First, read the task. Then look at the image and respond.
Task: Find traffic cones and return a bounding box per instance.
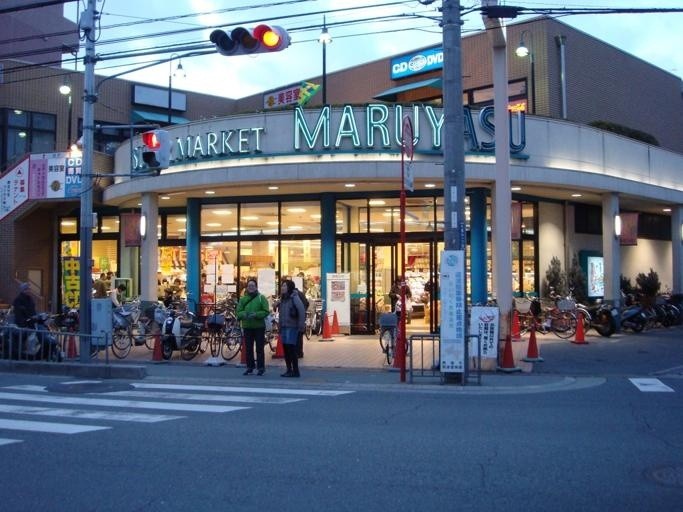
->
[495,334,521,373]
[318,311,336,343]
[569,311,590,345]
[330,309,344,337]
[518,329,545,364]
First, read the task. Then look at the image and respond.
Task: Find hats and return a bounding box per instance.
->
[21,282,32,289]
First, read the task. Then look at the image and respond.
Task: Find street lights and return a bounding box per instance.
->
[513,28,538,114]
[59,71,72,152]
[167,52,186,125]
[315,14,333,106]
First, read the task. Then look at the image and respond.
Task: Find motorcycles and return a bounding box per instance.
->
[567,281,682,338]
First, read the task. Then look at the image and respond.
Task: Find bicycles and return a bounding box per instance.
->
[304,299,322,340]
[378,303,409,366]
[486,285,592,342]
[110,302,279,361]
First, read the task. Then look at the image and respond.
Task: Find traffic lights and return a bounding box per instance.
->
[209,22,291,57]
[140,128,170,171]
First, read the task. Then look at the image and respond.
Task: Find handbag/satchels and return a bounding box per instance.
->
[290,293,309,319]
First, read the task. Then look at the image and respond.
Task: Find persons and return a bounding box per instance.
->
[271,279,306,379]
[92,268,305,307]
[386,275,413,320]
[10,282,37,326]
[235,279,265,376]
[291,279,309,358]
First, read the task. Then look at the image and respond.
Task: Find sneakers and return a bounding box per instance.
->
[280,370,301,378]
[242,369,265,377]
